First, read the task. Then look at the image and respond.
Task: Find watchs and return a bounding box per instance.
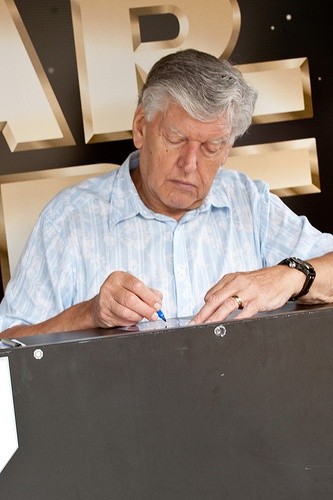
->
[275,256,316,302]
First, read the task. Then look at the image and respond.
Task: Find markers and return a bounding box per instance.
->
[1,337,22,348]
[126,270,167,323]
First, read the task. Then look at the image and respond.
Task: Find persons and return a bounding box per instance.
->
[0,47,333,342]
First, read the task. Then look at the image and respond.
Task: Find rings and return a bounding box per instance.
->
[231,294,243,309]
